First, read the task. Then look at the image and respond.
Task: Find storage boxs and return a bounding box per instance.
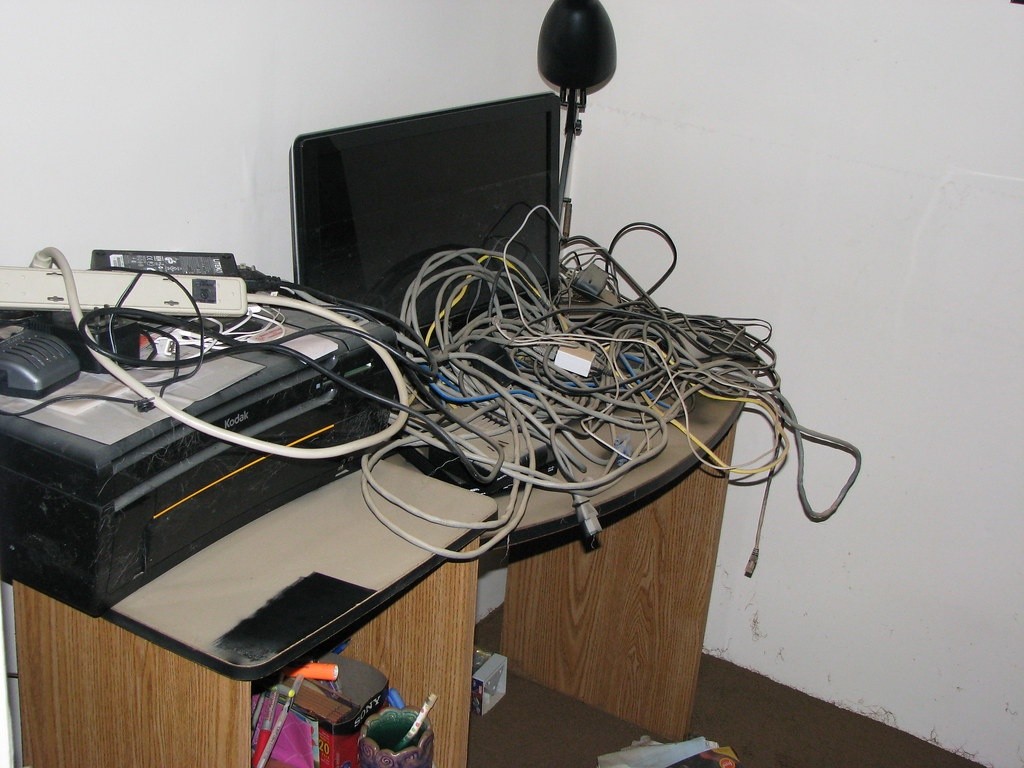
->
[470,646,507,716]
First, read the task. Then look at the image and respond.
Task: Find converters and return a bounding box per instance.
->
[91,250,243,278]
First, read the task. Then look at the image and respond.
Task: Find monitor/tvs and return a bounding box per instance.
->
[288,91,562,388]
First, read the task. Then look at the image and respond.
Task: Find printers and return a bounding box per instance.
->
[0,292,396,620]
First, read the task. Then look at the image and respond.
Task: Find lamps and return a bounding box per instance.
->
[537,0,620,310]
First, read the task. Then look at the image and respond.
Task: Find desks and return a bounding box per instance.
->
[12,361,759,768]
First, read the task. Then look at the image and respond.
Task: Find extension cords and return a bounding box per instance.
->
[0,265,247,318]
